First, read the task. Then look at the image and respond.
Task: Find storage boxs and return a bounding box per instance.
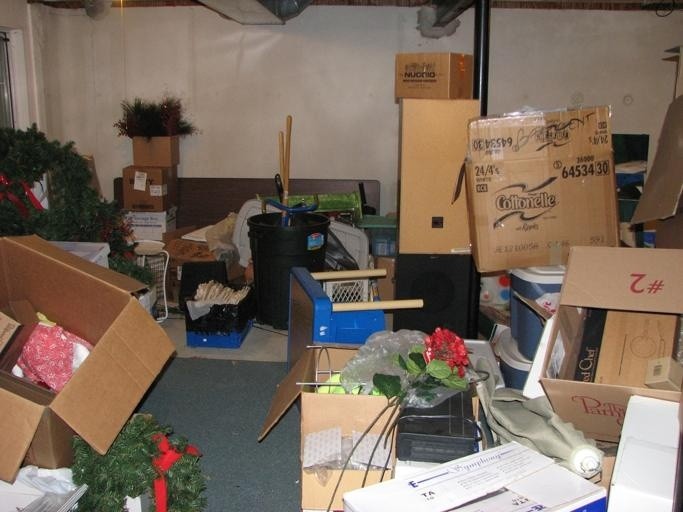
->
[392,51,474,102]
[0,233,177,484]
[121,136,254,348]
[397,95,482,255]
[464,104,622,275]
[261,344,403,512]
[539,246,682,444]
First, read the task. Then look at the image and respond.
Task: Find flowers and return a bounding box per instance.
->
[327,326,472,510]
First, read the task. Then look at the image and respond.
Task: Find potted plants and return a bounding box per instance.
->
[70,417,208,511]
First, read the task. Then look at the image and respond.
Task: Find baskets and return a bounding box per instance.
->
[324,277,369,305]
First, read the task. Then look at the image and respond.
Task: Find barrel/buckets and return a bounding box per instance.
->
[494,326,532,390]
[510,266,567,362]
[247,211,331,331]
